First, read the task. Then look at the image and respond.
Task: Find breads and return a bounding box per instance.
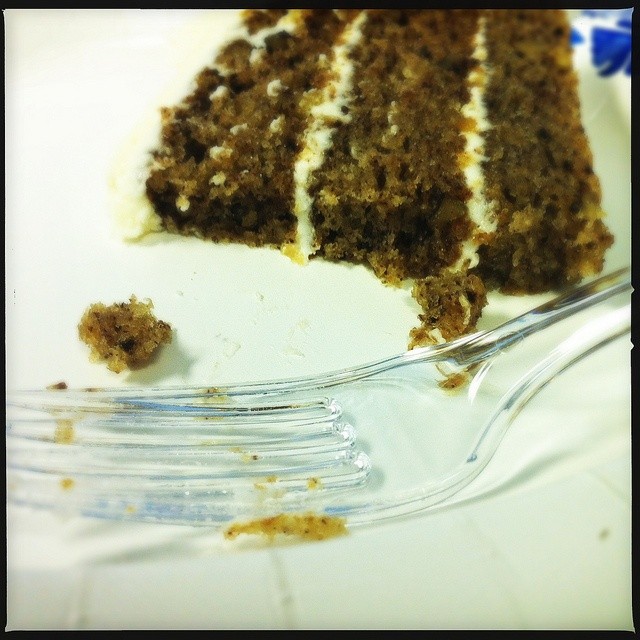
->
[142,8,615,394]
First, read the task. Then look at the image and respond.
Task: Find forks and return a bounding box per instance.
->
[3,266,630,539]
